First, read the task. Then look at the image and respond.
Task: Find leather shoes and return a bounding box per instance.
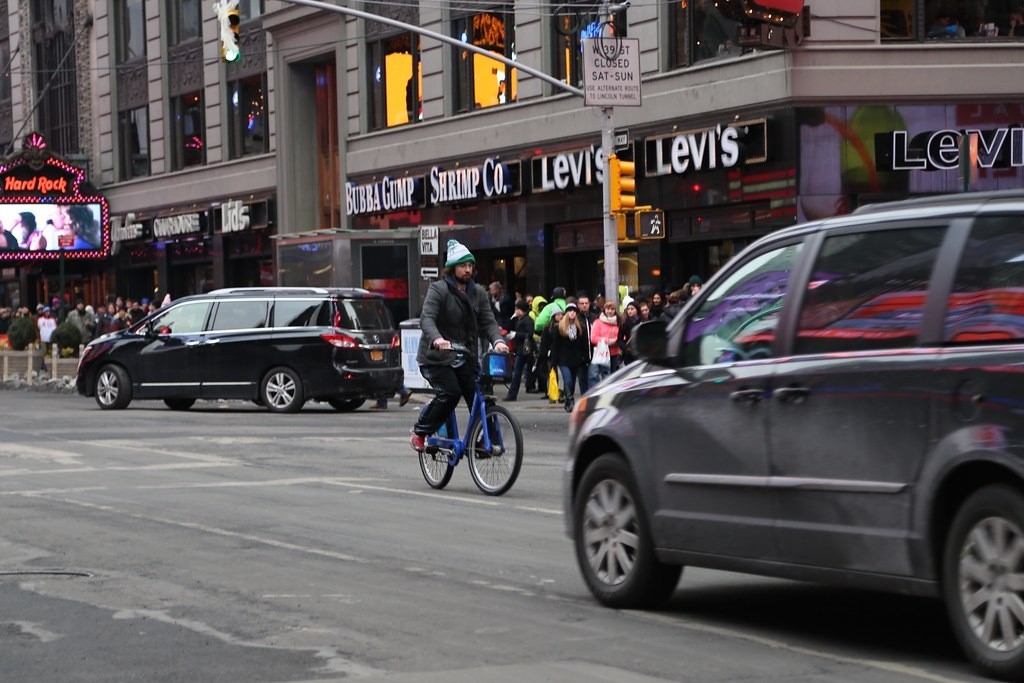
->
[369,403,388,410]
[399,391,412,407]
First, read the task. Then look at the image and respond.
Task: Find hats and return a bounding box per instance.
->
[690,274,702,286]
[565,302,579,313]
[445,239,476,265]
[551,307,563,318]
[515,300,529,312]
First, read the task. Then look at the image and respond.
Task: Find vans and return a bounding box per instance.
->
[561,188,1024,681]
[74,286,404,413]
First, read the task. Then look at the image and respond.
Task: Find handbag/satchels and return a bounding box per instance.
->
[548,367,565,402]
[591,339,612,368]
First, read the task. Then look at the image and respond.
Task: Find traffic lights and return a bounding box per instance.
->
[219,10,241,62]
[634,209,665,240]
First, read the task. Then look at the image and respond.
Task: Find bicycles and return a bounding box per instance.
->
[418,344,523,496]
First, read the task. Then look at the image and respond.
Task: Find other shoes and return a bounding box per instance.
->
[526,389,538,394]
[502,395,517,400]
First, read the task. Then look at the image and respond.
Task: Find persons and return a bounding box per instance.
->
[0,206,100,250]
[591,302,623,379]
[490,273,704,413]
[369,383,412,409]
[551,303,588,413]
[503,301,538,401]
[0,287,173,358]
[618,303,646,365]
[409,237,509,453]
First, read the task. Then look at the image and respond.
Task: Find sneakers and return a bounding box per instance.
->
[408,427,426,453]
